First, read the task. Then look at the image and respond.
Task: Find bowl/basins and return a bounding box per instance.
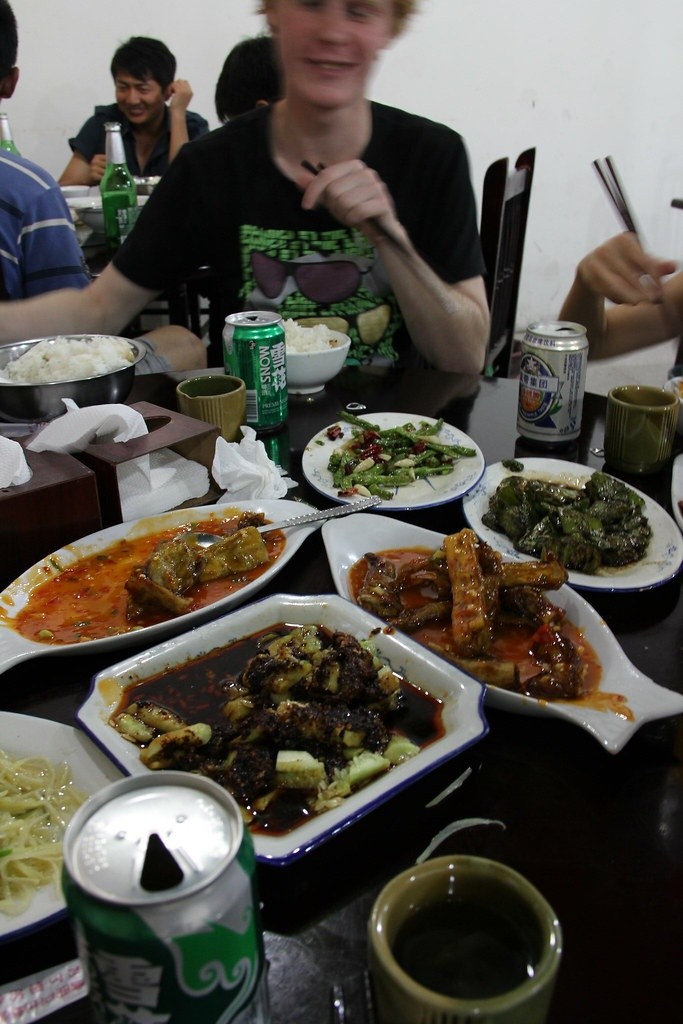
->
[284,329,351,394]
[0,335,148,426]
[662,376,683,434]
[59,176,161,237]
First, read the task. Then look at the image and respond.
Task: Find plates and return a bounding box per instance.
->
[302,413,487,511]
[319,512,683,754]
[0,500,327,678]
[671,454,683,534]
[75,593,490,867]
[462,457,683,592]
[0,711,127,942]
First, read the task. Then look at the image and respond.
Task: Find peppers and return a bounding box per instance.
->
[482,469,651,573]
[122,527,270,619]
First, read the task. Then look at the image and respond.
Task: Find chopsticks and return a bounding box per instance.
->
[593,155,674,338]
[300,159,411,257]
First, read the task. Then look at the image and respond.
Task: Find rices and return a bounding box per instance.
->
[283,318,343,353]
[0,335,133,382]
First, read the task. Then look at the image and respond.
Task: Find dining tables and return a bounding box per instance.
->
[0,369,681,1024]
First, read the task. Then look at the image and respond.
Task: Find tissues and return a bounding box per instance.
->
[36,397,213,517]
[0,426,103,584]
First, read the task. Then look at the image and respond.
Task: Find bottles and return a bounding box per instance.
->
[100,123,137,262]
[1,113,20,158]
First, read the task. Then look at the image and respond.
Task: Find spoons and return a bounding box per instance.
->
[173,496,381,549]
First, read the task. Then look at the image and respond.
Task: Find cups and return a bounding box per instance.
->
[368,855,564,1024]
[604,385,680,475]
[176,375,247,441]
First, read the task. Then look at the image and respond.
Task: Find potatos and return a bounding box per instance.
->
[0,748,93,918]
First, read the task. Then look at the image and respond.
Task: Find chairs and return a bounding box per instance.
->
[481,148,535,375]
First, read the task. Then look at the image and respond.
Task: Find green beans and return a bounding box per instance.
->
[329,410,478,501]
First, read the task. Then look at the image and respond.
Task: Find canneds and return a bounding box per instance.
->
[63,769,271,1024]
[516,320,588,442]
[220,310,289,432]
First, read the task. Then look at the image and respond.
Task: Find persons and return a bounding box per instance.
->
[215,35,286,123]
[0,0,208,375]
[0,0,493,381]
[57,37,211,187]
[558,196,683,403]
